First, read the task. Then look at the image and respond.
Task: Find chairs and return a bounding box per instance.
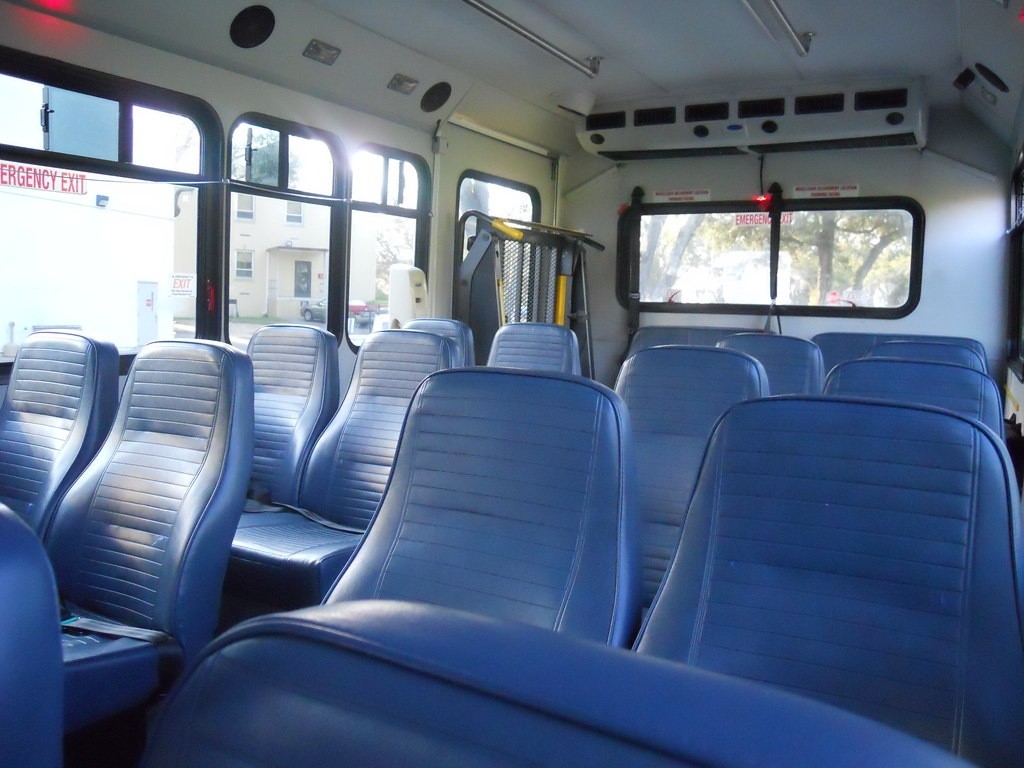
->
[1,318,1023,768]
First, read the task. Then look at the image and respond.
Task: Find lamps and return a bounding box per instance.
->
[96,194,109,207]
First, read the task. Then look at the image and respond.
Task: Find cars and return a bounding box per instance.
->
[300,296,389,327]
